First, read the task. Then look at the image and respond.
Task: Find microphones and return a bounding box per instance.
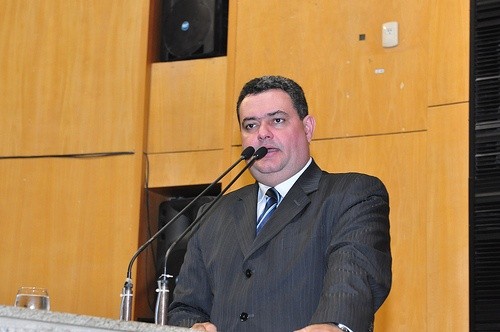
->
[154,147,268,327]
[119,146,255,321]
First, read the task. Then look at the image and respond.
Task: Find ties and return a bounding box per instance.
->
[256,188,281,237]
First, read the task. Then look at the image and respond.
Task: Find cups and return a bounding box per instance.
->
[14,287,50,311]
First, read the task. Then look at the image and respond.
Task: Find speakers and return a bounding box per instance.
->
[160,0,229,63]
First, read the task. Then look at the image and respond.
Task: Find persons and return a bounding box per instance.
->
[169,75,393,332]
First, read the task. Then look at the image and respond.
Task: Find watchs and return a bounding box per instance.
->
[335,323,354,332]
